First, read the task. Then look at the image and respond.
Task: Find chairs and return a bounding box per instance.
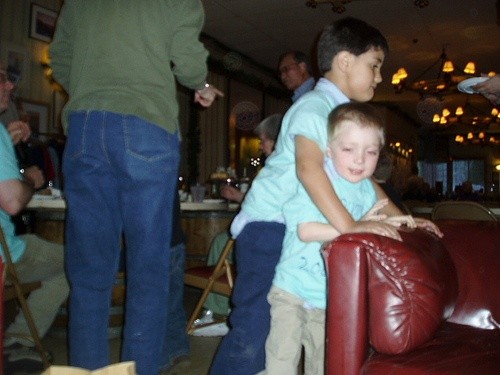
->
[433,201,498,220]
[182,238,236,332]
[0,227,48,375]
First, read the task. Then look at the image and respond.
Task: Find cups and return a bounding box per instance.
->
[190,186,206,202]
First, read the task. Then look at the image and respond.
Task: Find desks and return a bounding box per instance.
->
[24,200,239,337]
[413,198,500,217]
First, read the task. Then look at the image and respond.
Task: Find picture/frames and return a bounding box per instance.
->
[52,89,67,130]
[28,3,58,43]
[17,97,50,142]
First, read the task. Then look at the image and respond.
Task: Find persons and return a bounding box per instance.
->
[0,66,71,361]
[404,181,485,202]
[207,16,445,375]
[48,0,223,375]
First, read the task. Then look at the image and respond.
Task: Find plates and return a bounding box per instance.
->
[33,194,55,199]
[203,199,227,201]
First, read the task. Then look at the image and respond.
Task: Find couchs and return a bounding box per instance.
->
[325,220,500,375]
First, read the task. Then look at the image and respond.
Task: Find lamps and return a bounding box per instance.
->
[390,50,500,148]
